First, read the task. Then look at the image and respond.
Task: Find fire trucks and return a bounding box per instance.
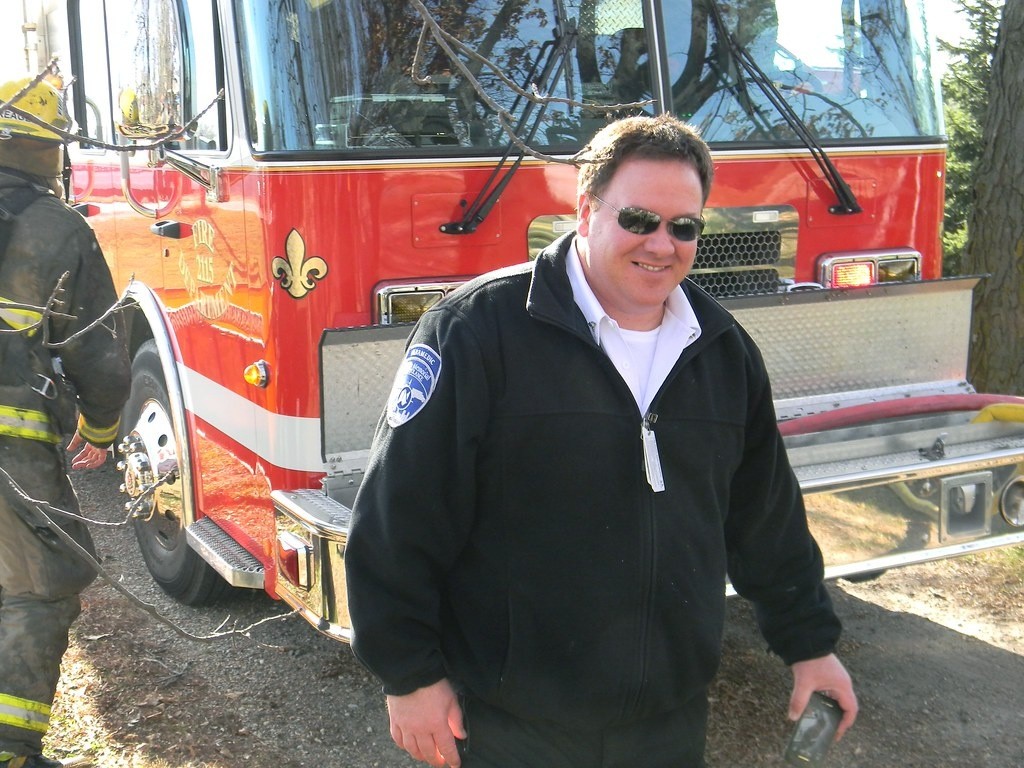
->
[2,1,1024,657]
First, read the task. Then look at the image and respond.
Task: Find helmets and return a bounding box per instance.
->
[0,76,69,142]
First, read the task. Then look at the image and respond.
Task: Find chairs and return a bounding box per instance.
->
[577,28,653,94]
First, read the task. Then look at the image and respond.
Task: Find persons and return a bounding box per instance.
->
[1,78,133,768]
[344,115,862,768]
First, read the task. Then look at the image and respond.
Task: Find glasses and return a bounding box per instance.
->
[592,192,706,241]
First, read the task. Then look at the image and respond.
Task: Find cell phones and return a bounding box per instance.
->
[783,692,843,768]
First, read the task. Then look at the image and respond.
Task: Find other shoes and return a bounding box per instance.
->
[0,749,63,768]
